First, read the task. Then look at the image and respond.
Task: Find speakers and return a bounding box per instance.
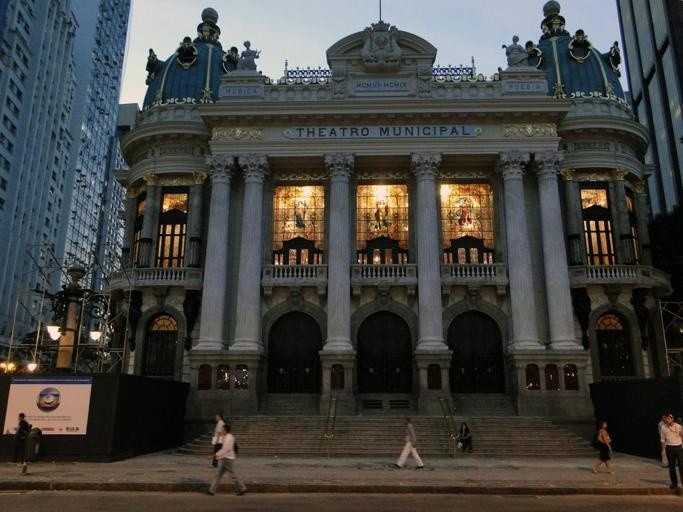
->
[592,465,597,473]
[606,471,613,474]
[416,466,423,469]
[237,490,248,496]
[207,465,216,468]
[392,463,401,470]
[204,490,214,496]
[670,484,677,489]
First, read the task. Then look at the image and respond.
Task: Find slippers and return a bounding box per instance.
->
[234,442,240,453]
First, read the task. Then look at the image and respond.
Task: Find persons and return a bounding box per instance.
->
[393,417,424,470]
[502,36,531,67]
[236,42,262,71]
[460,422,474,453]
[15,413,32,475]
[203,413,247,496]
[658,412,683,489]
[592,422,612,473]
